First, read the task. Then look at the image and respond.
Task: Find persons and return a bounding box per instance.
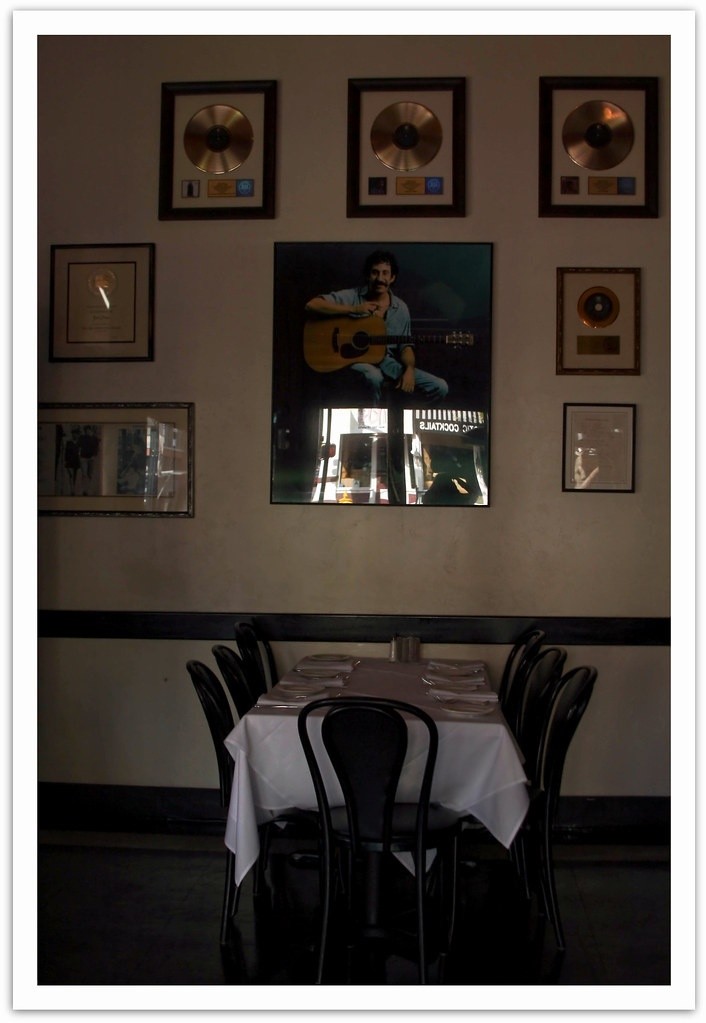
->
[63,426,98,497]
[120,428,168,498]
[304,252,449,400]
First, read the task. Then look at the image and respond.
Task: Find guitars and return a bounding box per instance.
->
[305,310,478,376]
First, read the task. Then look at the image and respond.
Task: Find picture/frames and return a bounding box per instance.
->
[537,76,660,219]
[37,401,195,520]
[555,266,642,377]
[47,243,157,364]
[158,80,276,221]
[560,402,638,495]
[269,238,496,509]
[346,77,465,219]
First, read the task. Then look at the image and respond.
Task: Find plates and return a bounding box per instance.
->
[308,653,352,660]
[277,682,325,695]
[296,667,341,677]
[421,660,494,714]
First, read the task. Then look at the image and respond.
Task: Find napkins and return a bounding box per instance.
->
[257,691,329,711]
[296,660,355,673]
[425,673,487,688]
[428,659,487,675]
[280,671,344,689]
[428,688,500,704]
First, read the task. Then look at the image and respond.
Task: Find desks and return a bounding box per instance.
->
[224,657,531,985]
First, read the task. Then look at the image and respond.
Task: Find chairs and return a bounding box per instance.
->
[187,620,598,985]
